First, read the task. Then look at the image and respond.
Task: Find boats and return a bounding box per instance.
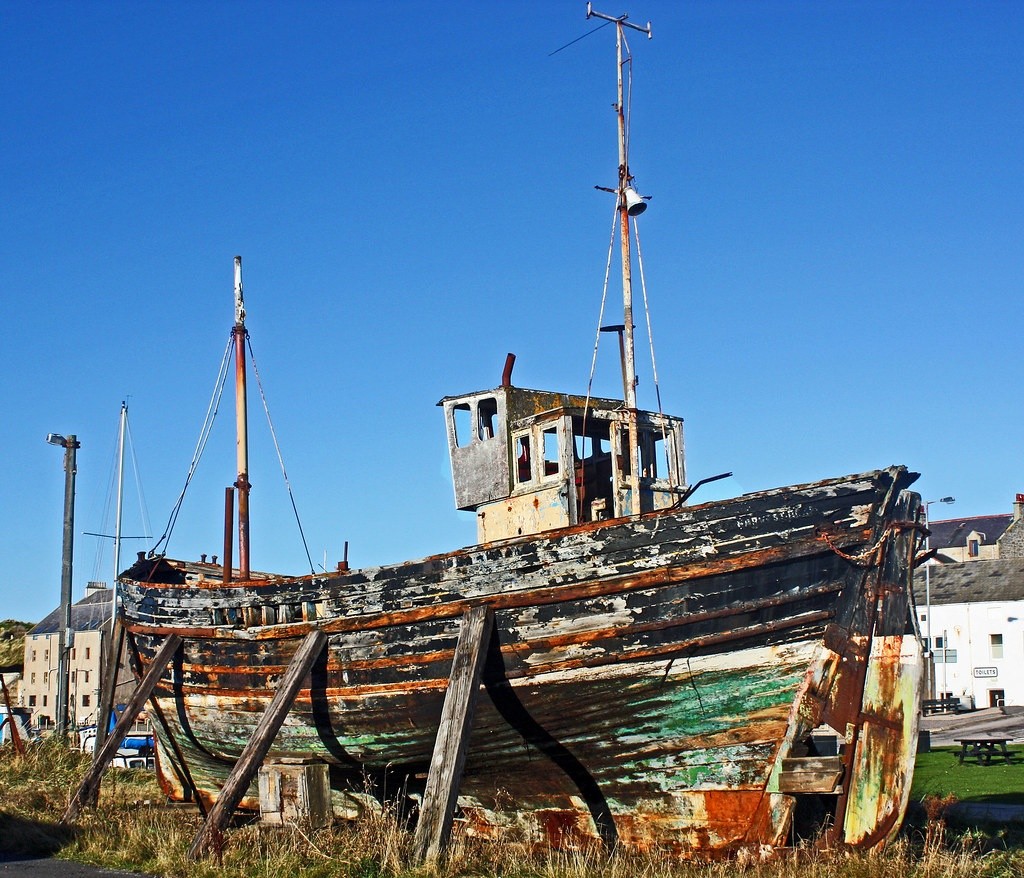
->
[79,725,155,769]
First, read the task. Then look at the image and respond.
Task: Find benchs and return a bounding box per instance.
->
[947,748,1021,756]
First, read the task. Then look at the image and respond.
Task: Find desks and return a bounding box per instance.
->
[953,737,1013,765]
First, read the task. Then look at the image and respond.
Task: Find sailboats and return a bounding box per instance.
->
[113,2,938,853]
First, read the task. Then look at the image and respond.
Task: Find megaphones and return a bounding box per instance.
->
[624,186,647,216]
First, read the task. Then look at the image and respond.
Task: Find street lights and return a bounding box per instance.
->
[46,433,81,739]
[925,496,955,715]
